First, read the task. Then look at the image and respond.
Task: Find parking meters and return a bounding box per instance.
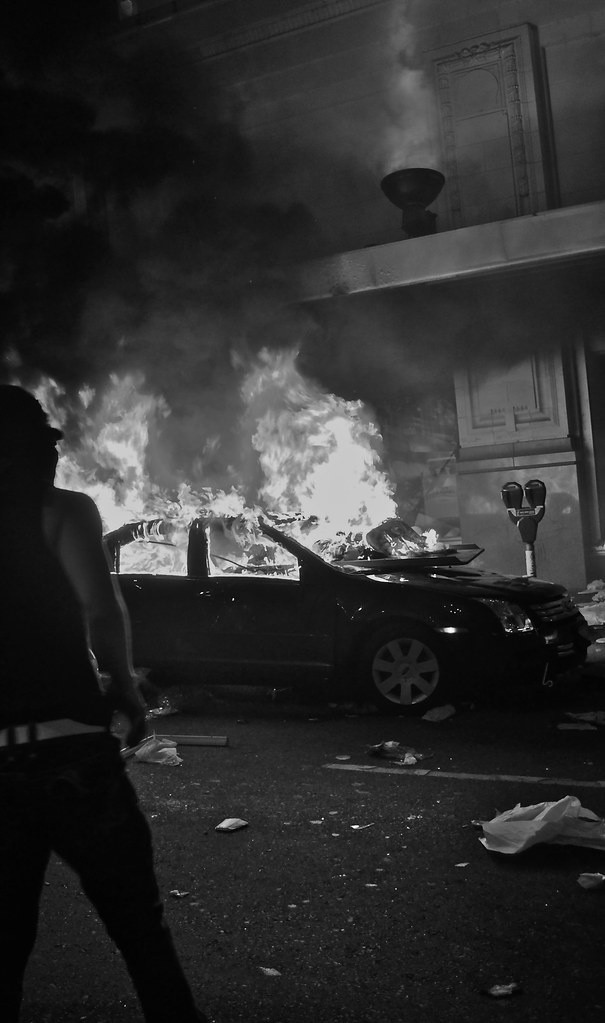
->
[499,482,548,579]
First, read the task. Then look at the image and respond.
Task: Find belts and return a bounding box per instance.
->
[0,717,109,746]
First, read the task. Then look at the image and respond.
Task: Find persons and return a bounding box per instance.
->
[0,384,215,1023]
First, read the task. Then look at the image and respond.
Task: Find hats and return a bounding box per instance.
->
[0,383,65,458]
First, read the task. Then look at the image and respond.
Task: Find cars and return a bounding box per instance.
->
[93,510,597,721]
